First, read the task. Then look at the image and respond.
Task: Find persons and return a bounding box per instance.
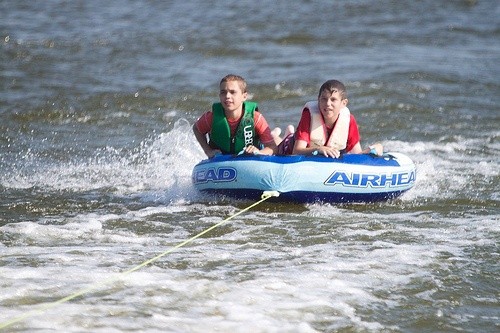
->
[283,80,383,158]
[192,74,275,156]
